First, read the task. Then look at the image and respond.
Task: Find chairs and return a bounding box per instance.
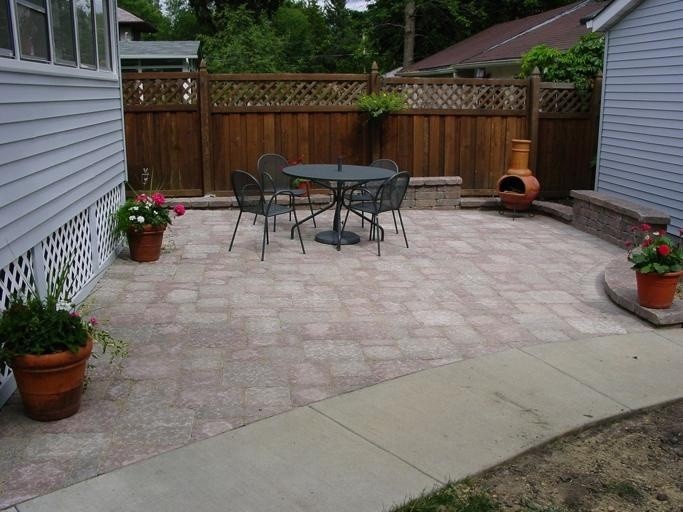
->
[228,152,410,257]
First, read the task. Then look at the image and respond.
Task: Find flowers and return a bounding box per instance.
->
[115,190,186,238]
[624,224,682,276]
[0,253,128,392]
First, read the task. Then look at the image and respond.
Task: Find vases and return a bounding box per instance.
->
[125,225,163,263]
[4,338,90,419]
[634,265,676,310]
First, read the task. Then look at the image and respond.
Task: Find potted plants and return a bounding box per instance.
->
[359,89,417,126]
